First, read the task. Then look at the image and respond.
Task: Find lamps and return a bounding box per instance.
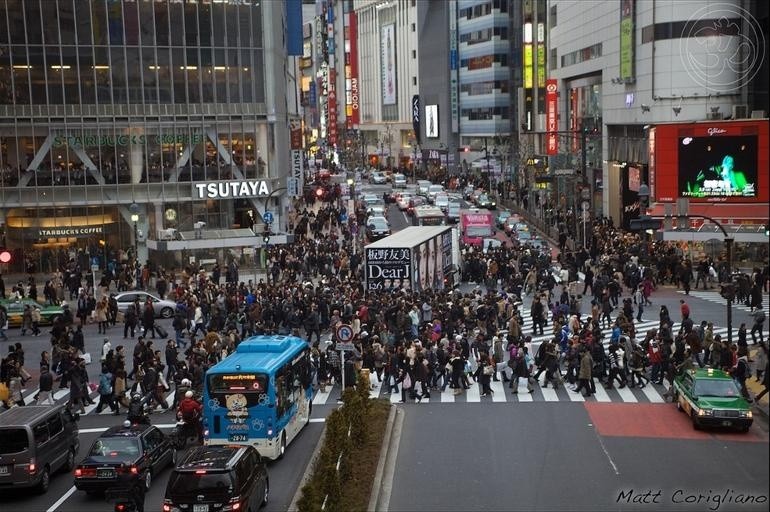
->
[640,99,660,113]
[708,96,720,112]
[673,96,683,115]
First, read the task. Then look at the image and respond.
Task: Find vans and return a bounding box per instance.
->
[0,404,80,494]
[162,444,271,512]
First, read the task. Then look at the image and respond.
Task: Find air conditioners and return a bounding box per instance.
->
[732,105,753,120]
[157,230,176,240]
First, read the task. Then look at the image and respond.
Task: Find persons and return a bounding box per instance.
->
[0,145,770,512]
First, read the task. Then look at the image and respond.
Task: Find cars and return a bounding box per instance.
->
[673,368,754,431]
[340,166,551,256]
[0,291,177,327]
[74,420,178,496]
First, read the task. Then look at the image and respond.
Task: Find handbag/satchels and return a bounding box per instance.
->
[79,352,91,365]
[483,360,495,375]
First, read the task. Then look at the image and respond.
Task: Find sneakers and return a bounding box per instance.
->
[440,382,471,395]
[400,400,405,402]
[493,378,510,382]
[605,377,649,389]
[65,399,96,414]
[471,375,494,397]
[509,383,534,394]
[652,378,663,385]
[574,387,596,397]
[534,377,563,388]
[379,379,399,395]
[409,386,439,400]
[111,412,119,416]
[21,332,42,336]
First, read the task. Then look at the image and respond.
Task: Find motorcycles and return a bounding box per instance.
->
[105,474,146,512]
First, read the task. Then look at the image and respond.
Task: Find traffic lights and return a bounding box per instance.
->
[262,231,270,242]
[311,186,325,197]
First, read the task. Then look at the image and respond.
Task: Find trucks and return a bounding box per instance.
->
[364,224,462,296]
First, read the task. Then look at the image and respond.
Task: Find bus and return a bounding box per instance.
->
[202,335,313,460]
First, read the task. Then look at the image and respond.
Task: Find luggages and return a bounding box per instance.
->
[154,325,168,339]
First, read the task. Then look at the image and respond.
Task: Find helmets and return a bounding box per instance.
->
[185,390,193,398]
[181,377,192,386]
[132,393,141,401]
